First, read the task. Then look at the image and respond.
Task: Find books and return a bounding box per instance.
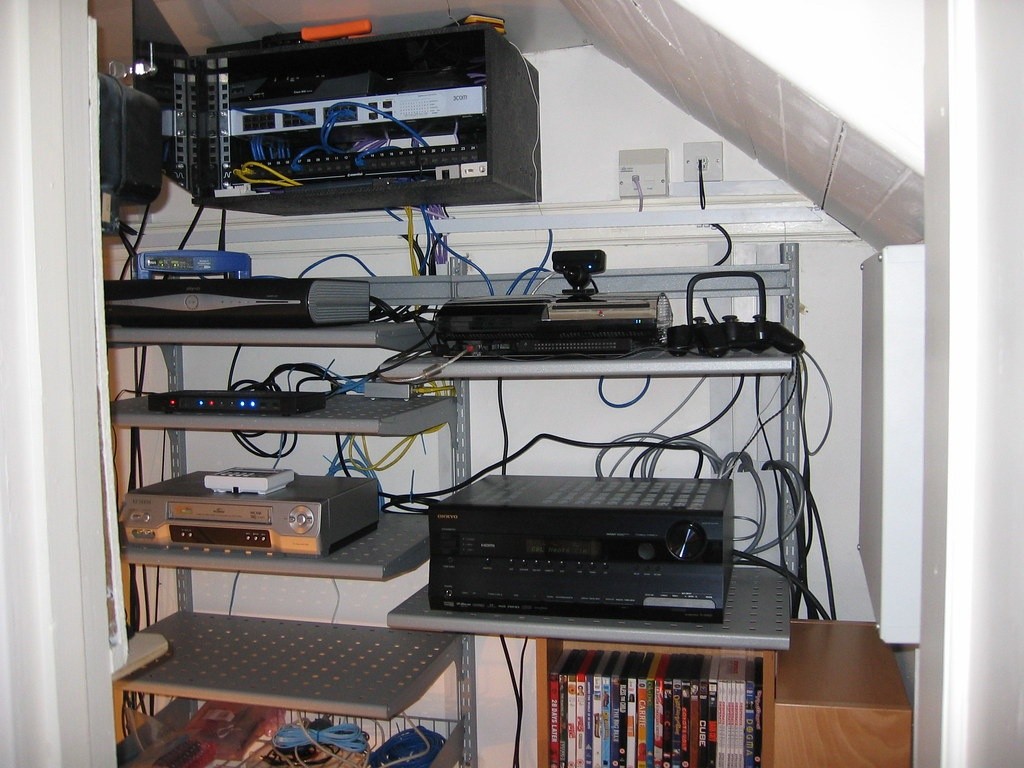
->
[549,649,763,768]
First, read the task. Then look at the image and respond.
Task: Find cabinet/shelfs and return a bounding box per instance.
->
[103,242,912,766]
[189,20,542,219]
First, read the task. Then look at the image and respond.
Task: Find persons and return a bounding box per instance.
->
[578,686,584,696]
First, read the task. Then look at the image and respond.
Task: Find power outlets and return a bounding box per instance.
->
[684,141,723,182]
